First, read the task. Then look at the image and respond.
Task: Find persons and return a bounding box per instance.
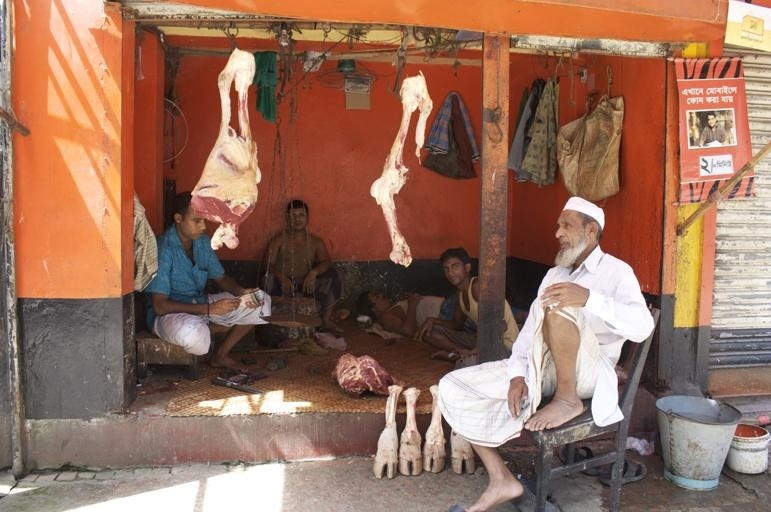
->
[697,110,729,148]
[434,194,657,512]
[256,197,344,349]
[410,245,521,370]
[353,286,529,338]
[140,189,272,372]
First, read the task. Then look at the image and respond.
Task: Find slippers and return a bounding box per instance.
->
[514,477,560,512]
[216,367,265,383]
[558,444,604,477]
[317,326,344,338]
[600,457,647,486]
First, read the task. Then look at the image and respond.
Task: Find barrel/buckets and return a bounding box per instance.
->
[656,394,743,490]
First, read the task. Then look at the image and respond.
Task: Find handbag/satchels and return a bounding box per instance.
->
[556,95,624,204]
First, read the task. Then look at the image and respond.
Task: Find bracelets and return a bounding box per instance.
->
[206,301,210,318]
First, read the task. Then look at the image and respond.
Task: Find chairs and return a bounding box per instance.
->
[500,290,662,512]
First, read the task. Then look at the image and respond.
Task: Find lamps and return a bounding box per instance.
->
[278,20,291,50]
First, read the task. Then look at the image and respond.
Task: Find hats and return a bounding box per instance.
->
[563,196,605,231]
